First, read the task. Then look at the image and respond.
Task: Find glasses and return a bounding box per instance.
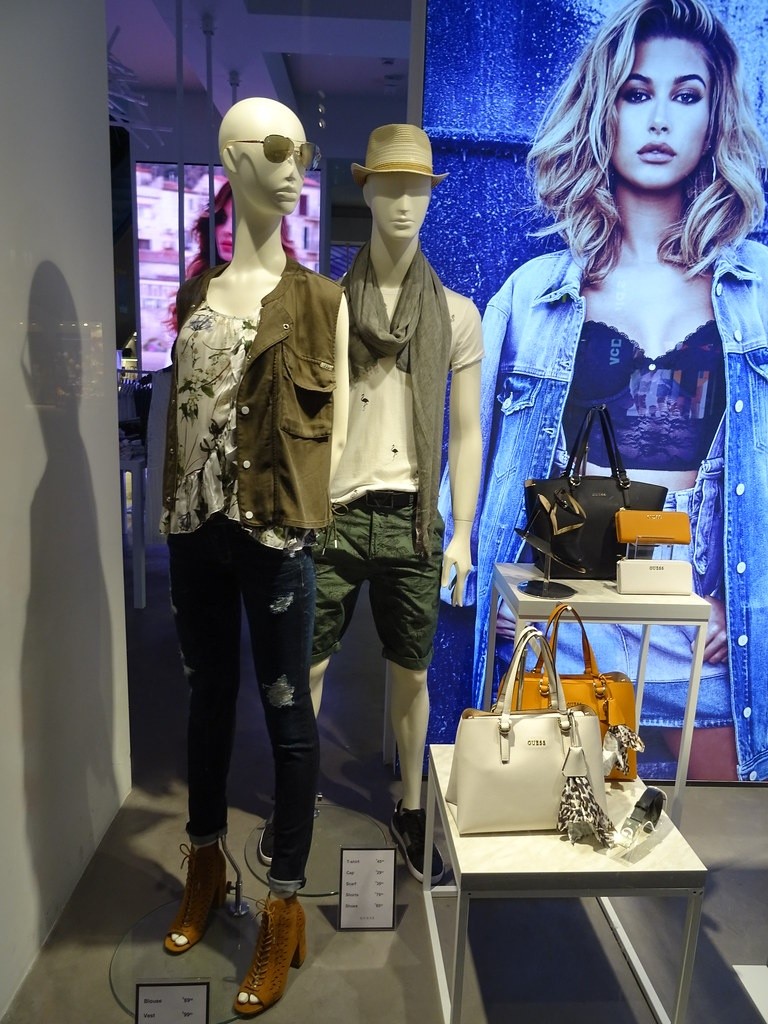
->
[223,134,321,173]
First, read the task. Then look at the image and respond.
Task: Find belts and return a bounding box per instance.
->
[616,786,664,849]
[355,490,417,511]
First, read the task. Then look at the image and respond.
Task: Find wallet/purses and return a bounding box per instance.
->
[616,557,692,596]
[615,510,690,545]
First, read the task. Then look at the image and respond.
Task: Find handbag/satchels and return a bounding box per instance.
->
[523,403,669,586]
[497,604,645,780]
[444,626,618,850]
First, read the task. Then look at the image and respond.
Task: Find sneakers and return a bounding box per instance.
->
[258,809,279,865]
[389,797,445,884]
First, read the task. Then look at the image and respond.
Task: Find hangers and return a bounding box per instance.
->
[116,368,153,397]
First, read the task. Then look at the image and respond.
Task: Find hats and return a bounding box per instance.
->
[350,124,450,188]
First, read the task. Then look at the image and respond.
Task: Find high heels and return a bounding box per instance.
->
[164,839,226,955]
[231,887,306,1018]
[523,488,587,569]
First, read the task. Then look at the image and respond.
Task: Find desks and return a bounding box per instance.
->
[423,744,708,1024]
[119,454,167,611]
[485,563,711,833]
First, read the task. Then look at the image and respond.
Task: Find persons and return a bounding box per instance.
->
[305,124,485,883]
[435,1,768,788]
[166,174,297,331]
[163,95,351,1015]
